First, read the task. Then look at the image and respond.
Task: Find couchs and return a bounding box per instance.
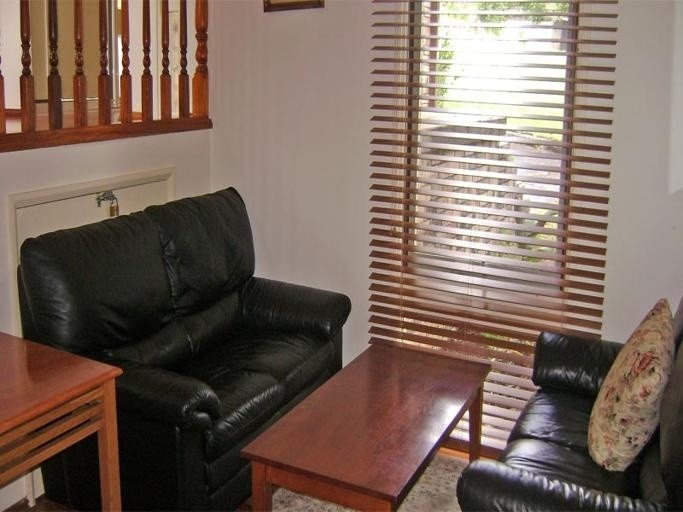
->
[456,297,682,512]
[16,186,351,512]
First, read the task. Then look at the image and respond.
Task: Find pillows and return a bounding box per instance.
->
[585,296,676,473]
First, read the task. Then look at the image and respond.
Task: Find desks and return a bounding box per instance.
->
[0,332,123,512]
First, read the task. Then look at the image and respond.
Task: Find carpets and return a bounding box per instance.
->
[268,450,470,512]
